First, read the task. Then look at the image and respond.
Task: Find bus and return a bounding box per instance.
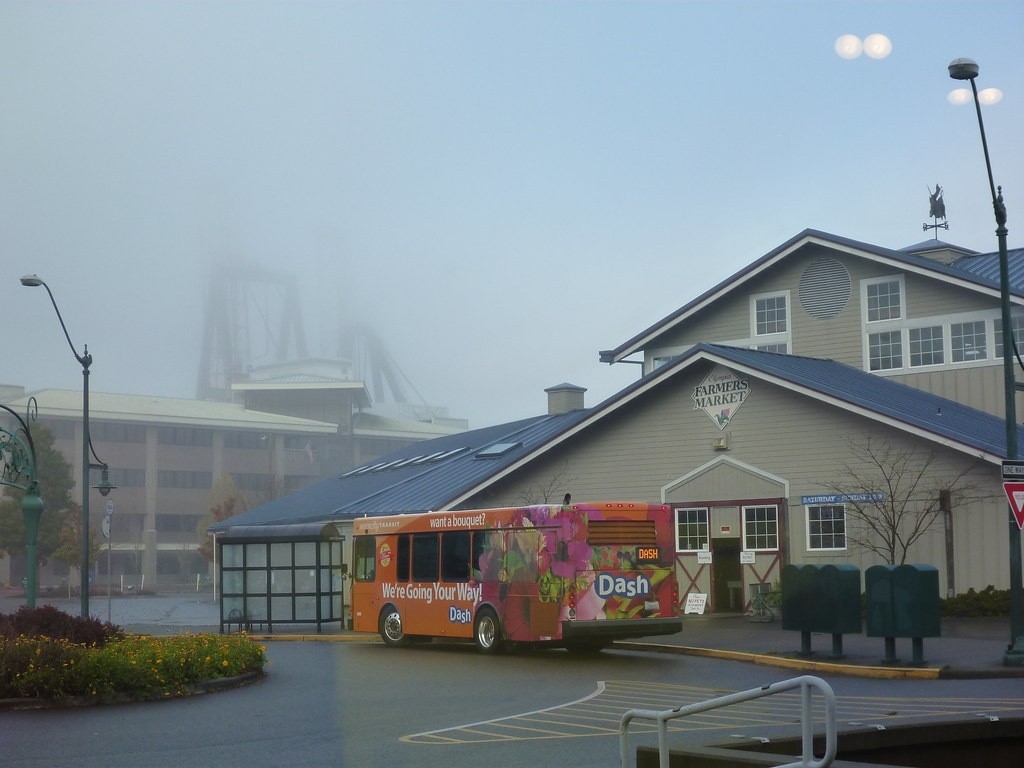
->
[341,491,684,654]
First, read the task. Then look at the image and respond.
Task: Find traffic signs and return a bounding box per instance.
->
[1002,459,1024,482]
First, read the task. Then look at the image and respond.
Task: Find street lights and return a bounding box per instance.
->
[135,529,157,589]
[19,272,119,620]
[948,55,1024,666]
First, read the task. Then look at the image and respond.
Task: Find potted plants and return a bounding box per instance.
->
[763,578,783,617]
